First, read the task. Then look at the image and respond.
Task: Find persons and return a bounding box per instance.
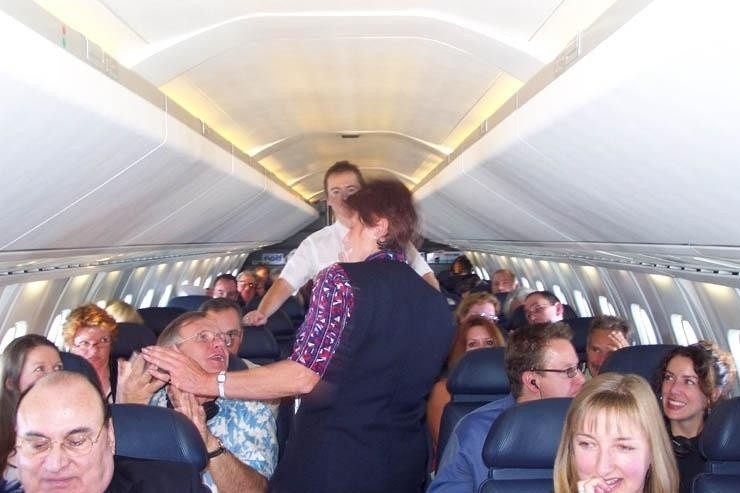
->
[552,373,681,493]
[115,266,304,493]
[141,177,460,493]
[0,301,141,493]
[662,339,736,493]
[430,255,634,462]
[427,324,587,493]
[242,161,442,328]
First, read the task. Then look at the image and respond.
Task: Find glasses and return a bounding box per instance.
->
[177,329,232,347]
[71,341,111,350]
[471,312,499,322]
[16,422,106,457]
[531,364,586,378]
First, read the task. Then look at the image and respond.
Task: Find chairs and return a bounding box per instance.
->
[433,271,579,336]
[477,397,576,490]
[241,306,296,359]
[63,351,107,432]
[107,405,214,493]
[108,322,157,402]
[553,317,596,369]
[597,343,689,398]
[693,395,740,490]
[435,346,511,475]
[237,325,280,367]
[282,296,303,330]
[136,307,188,341]
[227,355,285,484]
[166,294,213,311]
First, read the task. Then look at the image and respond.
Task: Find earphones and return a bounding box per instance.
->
[531,379,539,389]
